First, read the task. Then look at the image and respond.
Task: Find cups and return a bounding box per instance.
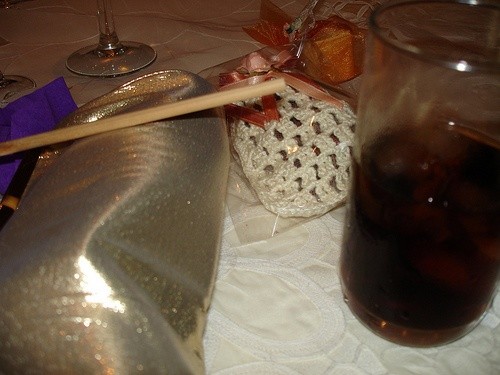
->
[336,2,499,346]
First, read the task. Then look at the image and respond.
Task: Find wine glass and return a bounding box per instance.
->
[66,2,156,77]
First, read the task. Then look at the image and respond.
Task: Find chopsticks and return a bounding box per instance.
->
[0,76,284,158]
